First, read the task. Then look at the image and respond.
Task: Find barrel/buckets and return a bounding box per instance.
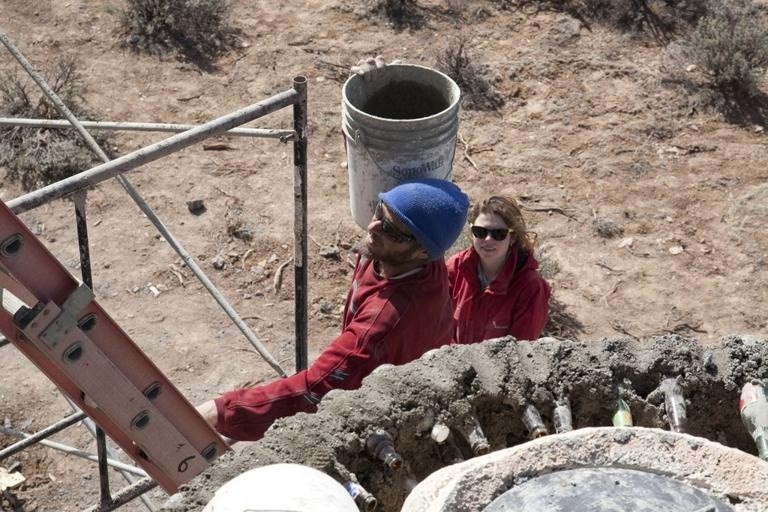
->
[342,64,460,232]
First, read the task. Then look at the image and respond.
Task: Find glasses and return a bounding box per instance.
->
[471,226,515,240]
[375,199,414,243]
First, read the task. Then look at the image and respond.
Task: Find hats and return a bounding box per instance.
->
[378,177,470,262]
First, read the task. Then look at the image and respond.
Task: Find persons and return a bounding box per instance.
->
[194,180,469,440]
[446,195,551,347]
